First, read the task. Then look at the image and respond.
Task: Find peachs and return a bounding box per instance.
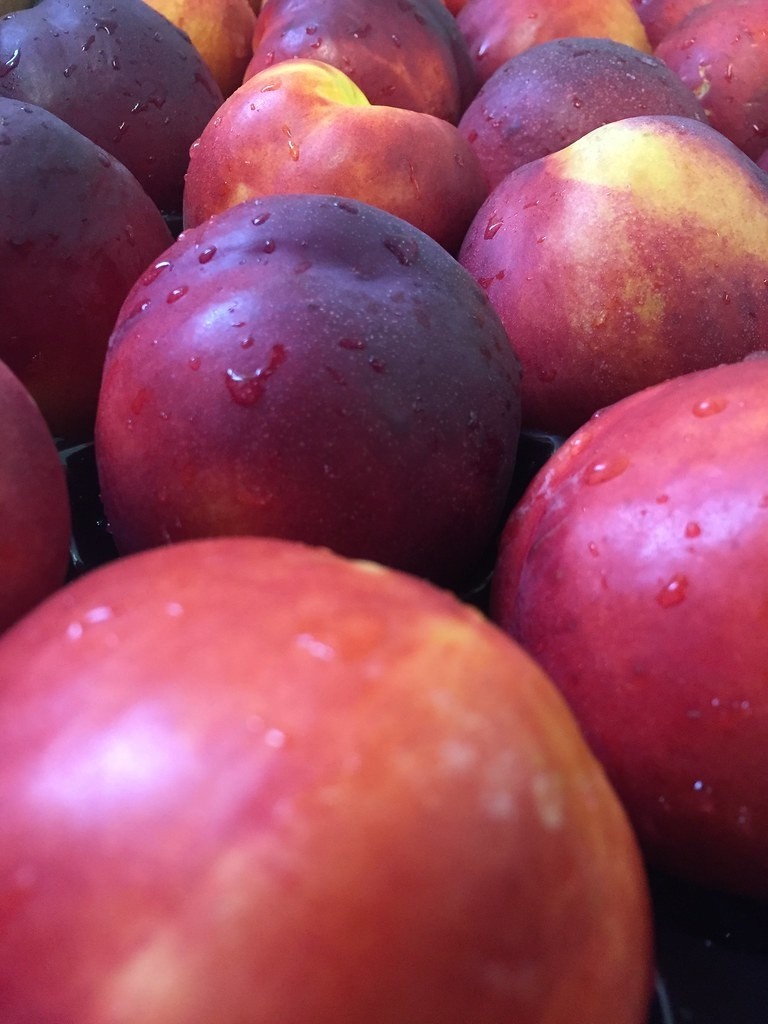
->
[0,0,767,1024]
